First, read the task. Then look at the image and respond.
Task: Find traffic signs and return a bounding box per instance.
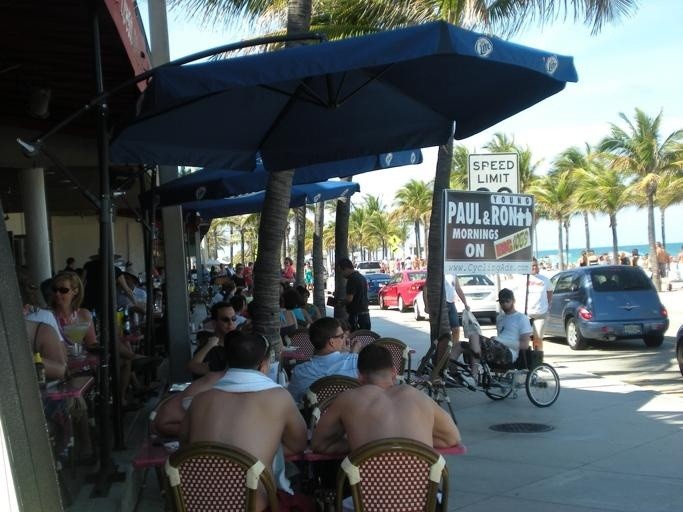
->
[468,152,518,194]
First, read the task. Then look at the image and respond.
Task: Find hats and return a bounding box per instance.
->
[497,288,515,302]
[123,269,139,280]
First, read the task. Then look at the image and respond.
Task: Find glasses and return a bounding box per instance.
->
[49,283,78,295]
[214,314,238,323]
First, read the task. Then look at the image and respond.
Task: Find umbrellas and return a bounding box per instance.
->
[87,149,430,448]
[130,176,360,400]
[17,19,575,492]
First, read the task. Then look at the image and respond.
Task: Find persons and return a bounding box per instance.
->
[514,257,551,372]
[444,289,530,390]
[538,241,683,297]
[16,248,471,510]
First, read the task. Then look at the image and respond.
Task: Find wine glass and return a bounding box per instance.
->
[63,323,89,359]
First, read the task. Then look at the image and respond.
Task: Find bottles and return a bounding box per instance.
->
[34,351,47,390]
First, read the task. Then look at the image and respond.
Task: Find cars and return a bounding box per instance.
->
[350,260,500,325]
[531,263,668,351]
[674,325,683,375]
[308,264,329,288]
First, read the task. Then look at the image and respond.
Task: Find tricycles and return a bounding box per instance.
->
[417,318,562,408]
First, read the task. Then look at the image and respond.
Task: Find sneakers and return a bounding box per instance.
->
[458,372,480,393]
